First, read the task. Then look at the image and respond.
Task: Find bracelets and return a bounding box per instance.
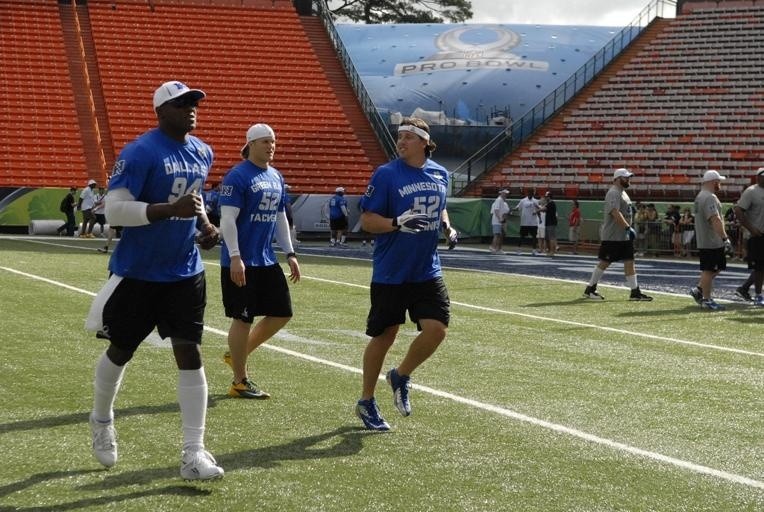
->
[287,253,297,259]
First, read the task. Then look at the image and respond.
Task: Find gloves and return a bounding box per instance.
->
[723,239,731,256]
[392,208,429,234]
[626,226,636,241]
[440,221,458,251]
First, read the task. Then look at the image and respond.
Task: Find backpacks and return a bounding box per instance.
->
[61,198,70,212]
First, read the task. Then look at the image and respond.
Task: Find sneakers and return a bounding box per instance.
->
[386,369,412,416]
[701,298,725,311]
[629,286,653,301]
[79,234,86,238]
[224,352,248,372]
[180,450,224,480]
[583,283,604,300]
[754,296,764,305]
[228,378,271,399]
[86,233,95,238]
[89,412,118,467]
[689,287,703,304]
[336,241,349,248]
[98,248,107,253]
[355,397,391,430]
[733,287,754,304]
[328,243,334,247]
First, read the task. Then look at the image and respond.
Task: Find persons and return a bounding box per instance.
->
[77,179,102,238]
[734,165,763,306]
[57,187,79,236]
[632,202,695,259]
[283,184,301,244]
[330,187,348,248]
[567,199,581,255]
[87,78,225,481]
[490,188,560,257]
[219,122,299,400]
[206,183,220,229]
[583,167,654,300]
[95,188,106,238]
[357,119,460,431]
[332,188,350,246]
[724,207,750,264]
[691,171,735,310]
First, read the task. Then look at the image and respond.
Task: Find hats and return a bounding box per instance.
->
[544,192,552,197]
[153,81,206,113]
[703,171,726,182]
[614,168,633,180]
[336,187,345,192]
[88,180,96,186]
[71,186,77,190]
[646,204,654,207]
[498,189,510,194]
[757,168,764,176]
[284,184,292,188]
[241,124,275,158]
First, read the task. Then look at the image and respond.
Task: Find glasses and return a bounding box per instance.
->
[166,97,195,109]
